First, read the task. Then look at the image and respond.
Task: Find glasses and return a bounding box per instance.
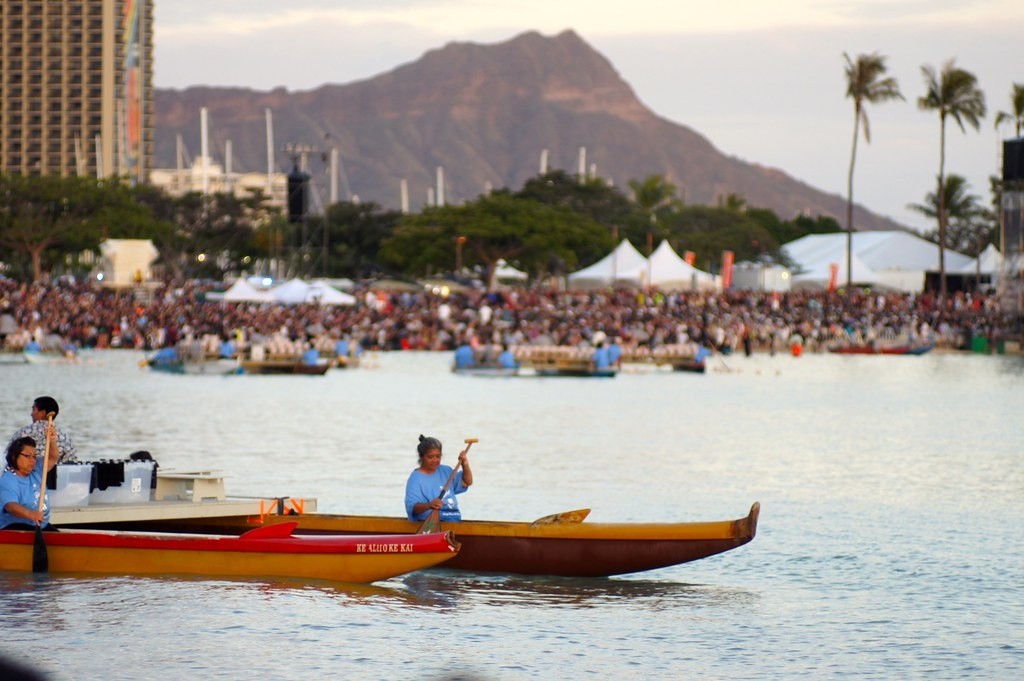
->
[20,452,38,459]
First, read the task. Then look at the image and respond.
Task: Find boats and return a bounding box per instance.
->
[21,353,81,365]
[0,521,462,585]
[141,338,714,376]
[827,339,934,356]
[206,503,760,575]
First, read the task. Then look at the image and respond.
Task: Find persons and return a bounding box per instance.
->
[1,423,60,534]
[405,434,475,523]
[7,395,78,492]
[0,260,1023,374]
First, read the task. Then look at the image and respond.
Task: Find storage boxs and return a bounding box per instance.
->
[88,459,155,504]
[49,464,94,510]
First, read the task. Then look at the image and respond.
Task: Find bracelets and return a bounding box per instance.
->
[461,459,468,465]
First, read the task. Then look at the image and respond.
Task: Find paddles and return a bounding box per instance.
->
[32,411,55,573]
[415,438,478,535]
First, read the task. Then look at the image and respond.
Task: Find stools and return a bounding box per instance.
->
[155,473,225,503]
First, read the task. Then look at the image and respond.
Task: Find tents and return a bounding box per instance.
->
[566,227,1023,295]
